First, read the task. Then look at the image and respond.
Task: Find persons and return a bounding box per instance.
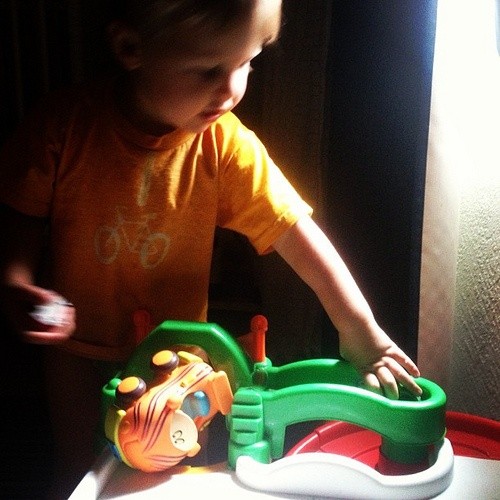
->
[1,0,422,500]
